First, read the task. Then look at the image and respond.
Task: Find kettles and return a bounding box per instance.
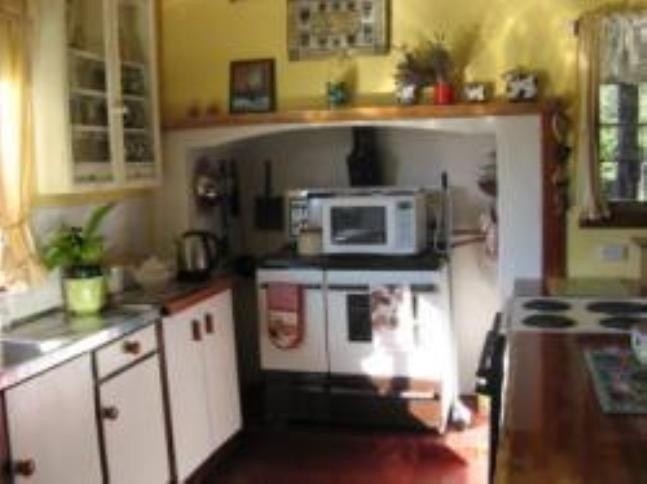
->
[174,228,220,282]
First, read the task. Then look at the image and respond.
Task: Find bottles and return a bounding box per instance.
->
[298,224,321,255]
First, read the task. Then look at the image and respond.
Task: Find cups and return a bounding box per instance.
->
[102,257,172,294]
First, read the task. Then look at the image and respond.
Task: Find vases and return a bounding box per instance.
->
[502,76,542,103]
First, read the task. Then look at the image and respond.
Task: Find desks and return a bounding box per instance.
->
[487,331,647,484]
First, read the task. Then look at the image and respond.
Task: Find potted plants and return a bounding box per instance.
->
[393,28,457,106]
[40,195,116,322]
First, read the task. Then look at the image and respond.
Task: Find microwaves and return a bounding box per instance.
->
[320,192,427,257]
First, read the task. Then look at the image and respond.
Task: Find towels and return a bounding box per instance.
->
[266,281,302,350]
[369,280,416,371]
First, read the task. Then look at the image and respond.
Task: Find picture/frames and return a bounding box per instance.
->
[225,56,277,113]
[284,0,391,62]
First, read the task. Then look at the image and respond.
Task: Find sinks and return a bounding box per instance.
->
[0,338,70,373]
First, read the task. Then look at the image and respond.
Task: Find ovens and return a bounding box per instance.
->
[323,270,443,397]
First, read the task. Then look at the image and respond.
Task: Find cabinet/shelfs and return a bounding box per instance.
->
[94,322,176,482]
[24,0,164,199]
[0,351,107,482]
[158,288,246,482]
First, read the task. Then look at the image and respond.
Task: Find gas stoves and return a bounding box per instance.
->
[504,295,647,335]
